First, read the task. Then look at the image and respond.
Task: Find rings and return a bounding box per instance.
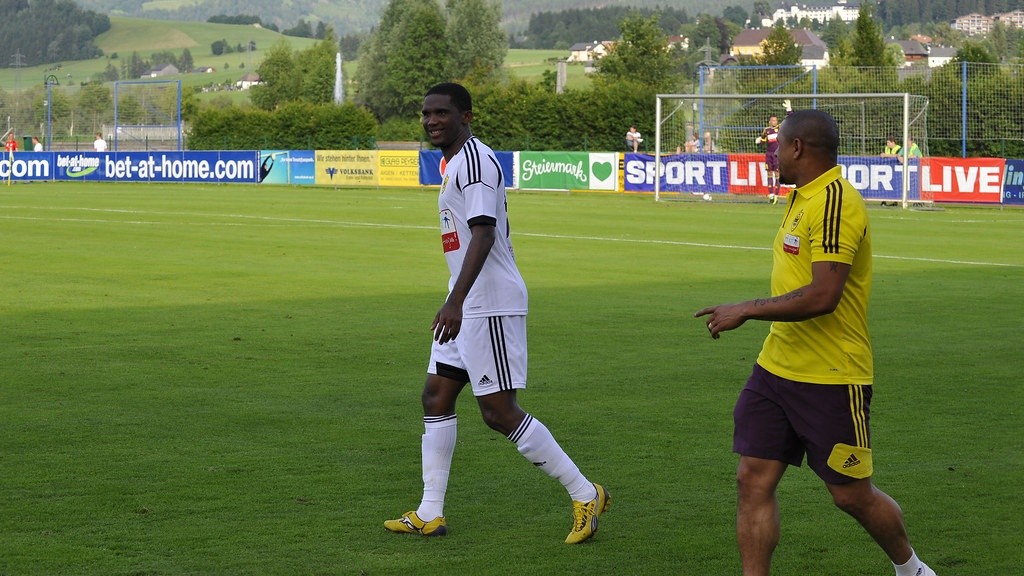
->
[708,323,713,329]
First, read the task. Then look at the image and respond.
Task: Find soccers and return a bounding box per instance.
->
[702,193,712,201]
[271,153,277,161]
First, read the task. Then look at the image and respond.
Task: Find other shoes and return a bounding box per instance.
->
[890,202,898,206]
[769,195,774,205]
[774,196,779,205]
[881,201,886,206]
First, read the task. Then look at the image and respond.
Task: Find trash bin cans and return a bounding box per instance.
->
[23,135,33,151]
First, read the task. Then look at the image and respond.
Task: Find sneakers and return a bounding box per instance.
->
[384,511,447,536]
[565,483,611,544]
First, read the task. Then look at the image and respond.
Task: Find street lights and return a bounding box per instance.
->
[46,74,59,151]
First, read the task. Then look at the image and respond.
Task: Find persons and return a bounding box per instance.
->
[896,135,923,207]
[880,136,901,207]
[626,125,643,152]
[756,100,793,205]
[694,109,936,576]
[94,132,107,152]
[691,132,714,154]
[383,84,611,545]
[32,136,43,152]
[5,132,18,151]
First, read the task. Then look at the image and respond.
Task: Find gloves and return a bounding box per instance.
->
[755,137,762,144]
[782,100,792,112]
